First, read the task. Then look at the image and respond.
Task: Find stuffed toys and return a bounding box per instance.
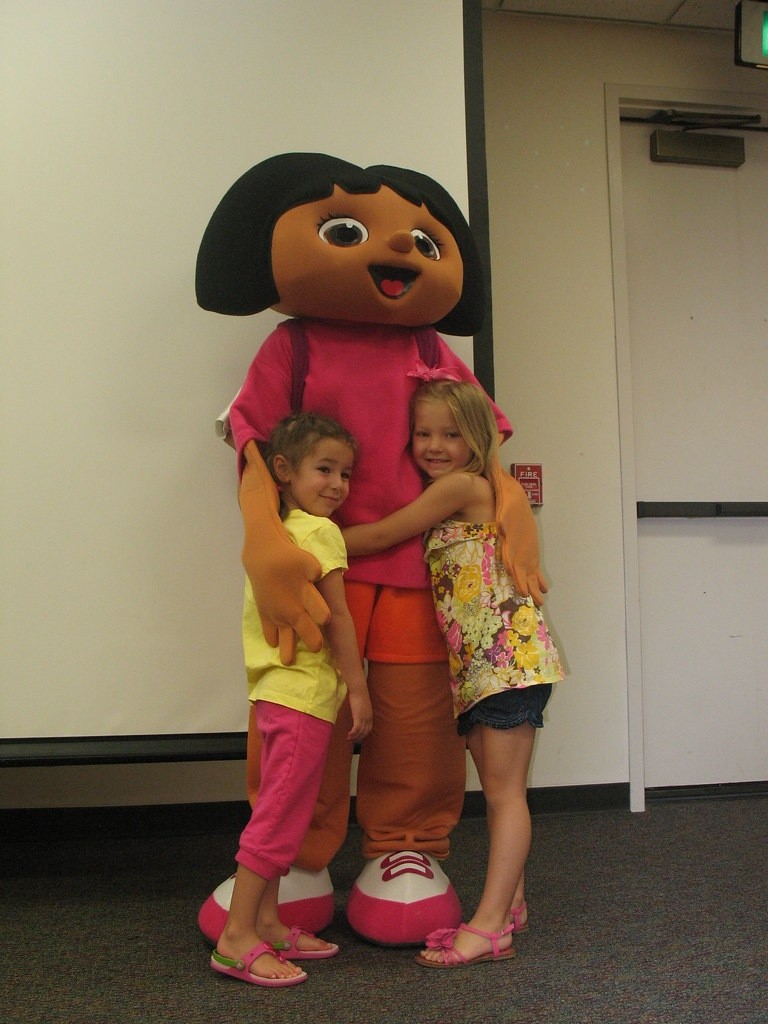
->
[195,152,548,947]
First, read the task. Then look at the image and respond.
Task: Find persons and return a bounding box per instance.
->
[211,413,374,987]
[344,379,564,969]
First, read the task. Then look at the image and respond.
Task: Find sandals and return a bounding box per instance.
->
[266,927,338,960]
[210,942,309,987]
[509,901,528,934]
[413,924,515,968]
